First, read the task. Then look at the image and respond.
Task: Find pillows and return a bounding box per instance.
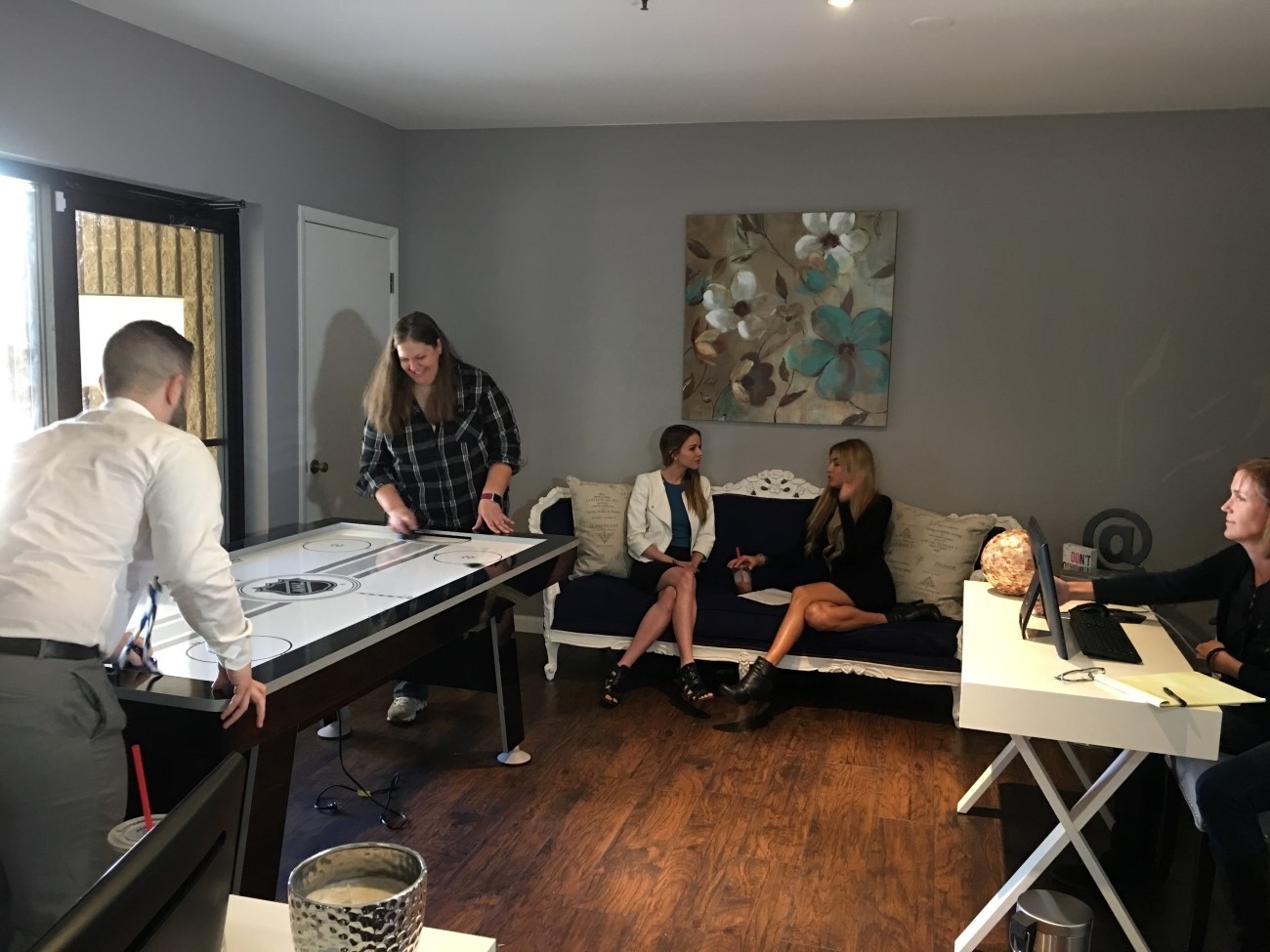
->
[566,476,633,580]
[881,499,998,621]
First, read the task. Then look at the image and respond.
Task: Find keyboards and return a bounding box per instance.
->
[1069,610,1143,663]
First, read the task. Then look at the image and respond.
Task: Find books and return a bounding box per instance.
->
[1095,671,1266,710]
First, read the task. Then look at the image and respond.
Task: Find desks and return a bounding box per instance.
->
[98,517,583,903]
[950,576,1224,952]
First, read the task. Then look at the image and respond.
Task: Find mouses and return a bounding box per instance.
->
[1070,603,1110,616]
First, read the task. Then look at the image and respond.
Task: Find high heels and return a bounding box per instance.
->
[602,662,630,706]
[882,599,942,624]
[673,659,715,701]
[720,656,777,705]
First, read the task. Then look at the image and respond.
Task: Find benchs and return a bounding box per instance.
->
[526,469,1022,731]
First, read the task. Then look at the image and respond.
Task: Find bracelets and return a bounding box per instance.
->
[1205,647,1228,675]
[672,558,676,565]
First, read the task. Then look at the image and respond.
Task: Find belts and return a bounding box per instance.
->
[0,636,100,660]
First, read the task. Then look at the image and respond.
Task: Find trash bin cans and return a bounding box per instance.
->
[1010,888,1095,952]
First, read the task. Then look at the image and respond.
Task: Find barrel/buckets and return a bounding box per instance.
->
[1006,889,1096,951]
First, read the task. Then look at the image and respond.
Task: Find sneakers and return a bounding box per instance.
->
[388,695,427,724]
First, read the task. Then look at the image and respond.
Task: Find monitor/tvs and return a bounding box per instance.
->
[1019,515,1069,662]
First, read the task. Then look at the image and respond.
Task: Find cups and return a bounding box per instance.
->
[107,814,167,861]
[731,562,751,594]
[288,843,428,952]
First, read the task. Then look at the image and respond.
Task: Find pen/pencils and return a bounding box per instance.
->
[1163,686,1187,708]
[736,547,747,583]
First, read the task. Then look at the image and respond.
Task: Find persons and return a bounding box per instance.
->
[0,321,267,952]
[354,311,521,723]
[1034,457,1270,952]
[716,439,944,708]
[601,424,716,707]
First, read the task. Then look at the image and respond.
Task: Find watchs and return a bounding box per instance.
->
[480,493,502,509]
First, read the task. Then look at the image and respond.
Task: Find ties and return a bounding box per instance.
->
[118,576,160,673]
